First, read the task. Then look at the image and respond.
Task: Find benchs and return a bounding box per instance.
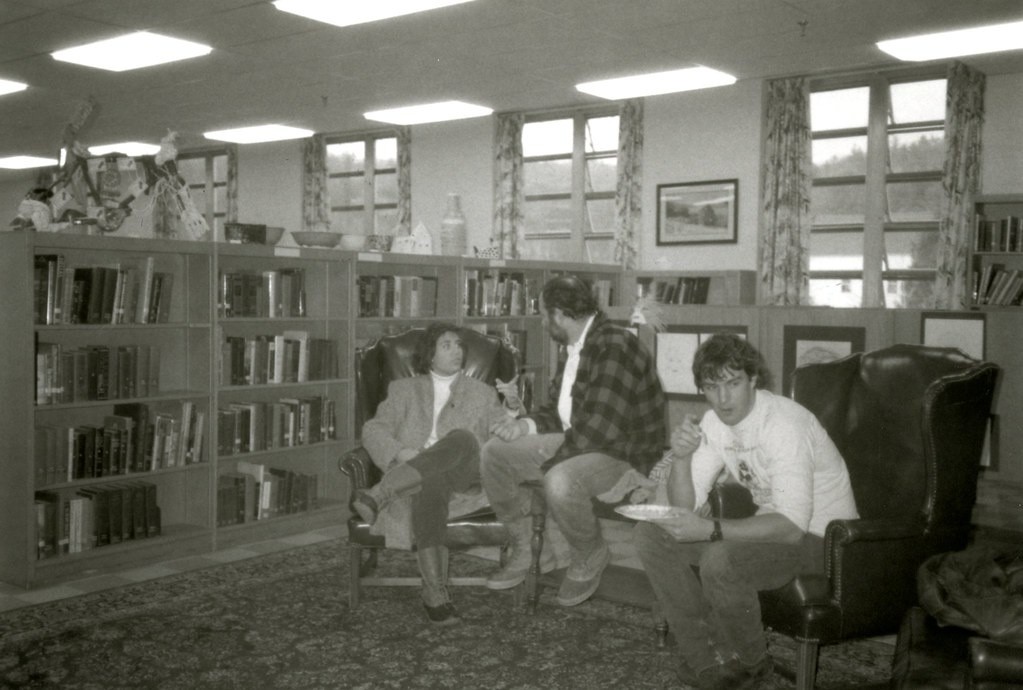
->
[519,479,670,650]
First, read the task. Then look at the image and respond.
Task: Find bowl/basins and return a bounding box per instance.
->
[224,222,394,252]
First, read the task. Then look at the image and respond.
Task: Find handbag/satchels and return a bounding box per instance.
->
[707,482,756,520]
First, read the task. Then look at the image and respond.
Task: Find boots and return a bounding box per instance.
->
[557,520,611,606]
[353,465,422,526]
[486,516,557,590]
[418,545,463,625]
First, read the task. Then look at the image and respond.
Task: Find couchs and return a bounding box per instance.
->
[888,604,1023,690]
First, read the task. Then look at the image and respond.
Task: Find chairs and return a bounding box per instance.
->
[338,316,514,611]
[693,341,1001,690]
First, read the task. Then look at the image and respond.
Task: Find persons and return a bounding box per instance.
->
[632,334,860,690]
[480,275,663,604]
[353,322,527,622]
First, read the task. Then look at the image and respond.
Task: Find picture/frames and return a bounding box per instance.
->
[655,178,739,247]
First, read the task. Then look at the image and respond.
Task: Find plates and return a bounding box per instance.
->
[614,503,679,521]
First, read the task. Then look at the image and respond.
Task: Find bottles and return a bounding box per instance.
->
[441,193,467,256]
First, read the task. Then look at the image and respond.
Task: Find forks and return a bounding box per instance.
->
[691,421,707,445]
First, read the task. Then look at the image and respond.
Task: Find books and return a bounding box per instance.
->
[217,461,318,528]
[220,265,307,316]
[469,322,535,410]
[217,394,336,455]
[972,263,1023,306]
[35,401,205,484]
[357,275,436,317]
[464,268,540,314]
[217,325,338,384]
[553,271,619,307]
[35,482,162,559]
[637,279,710,304]
[34,254,173,324]
[974,213,1023,252]
[34,331,161,406]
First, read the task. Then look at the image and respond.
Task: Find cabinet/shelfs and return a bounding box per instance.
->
[0,229,624,614]
[620,269,755,308]
[963,194,1023,312]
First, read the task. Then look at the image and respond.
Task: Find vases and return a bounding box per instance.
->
[439,193,468,258]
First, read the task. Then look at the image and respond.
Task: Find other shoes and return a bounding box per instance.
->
[677,659,774,690]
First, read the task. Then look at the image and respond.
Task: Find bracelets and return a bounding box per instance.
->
[710,520,723,541]
